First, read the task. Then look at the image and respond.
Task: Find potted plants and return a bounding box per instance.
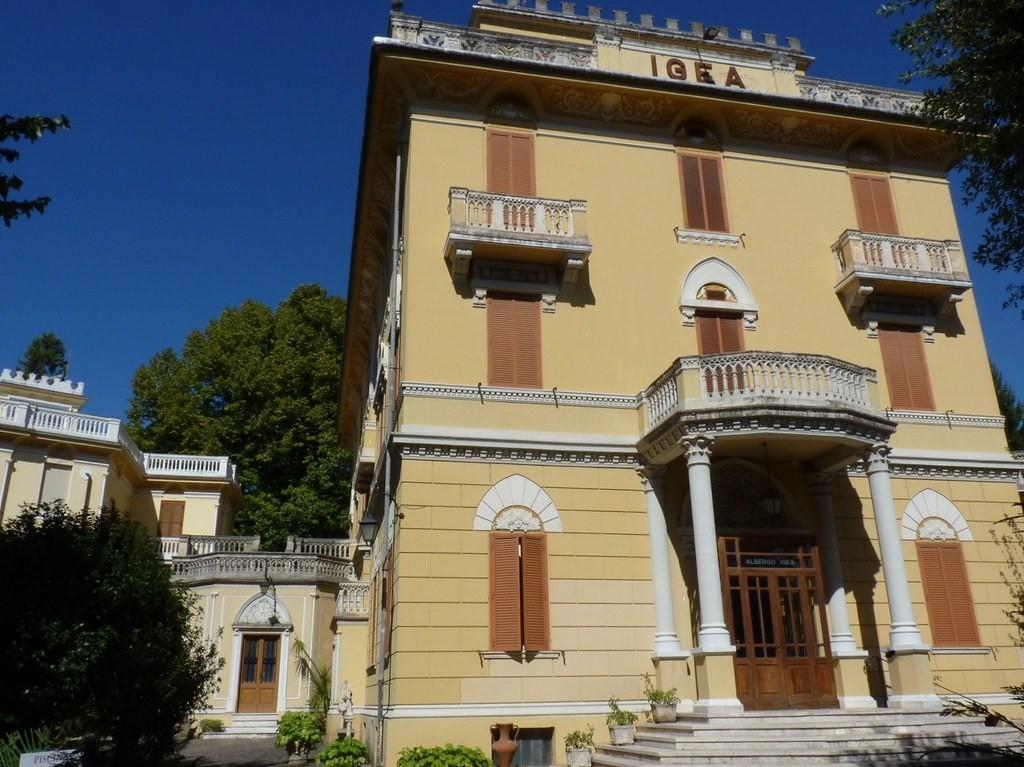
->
[640,671,682,723]
[274,708,326,766]
[564,723,597,767]
[606,694,640,747]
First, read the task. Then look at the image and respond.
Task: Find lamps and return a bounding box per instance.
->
[358,491,404,547]
[702,26,720,40]
[257,576,272,595]
[758,441,784,523]
